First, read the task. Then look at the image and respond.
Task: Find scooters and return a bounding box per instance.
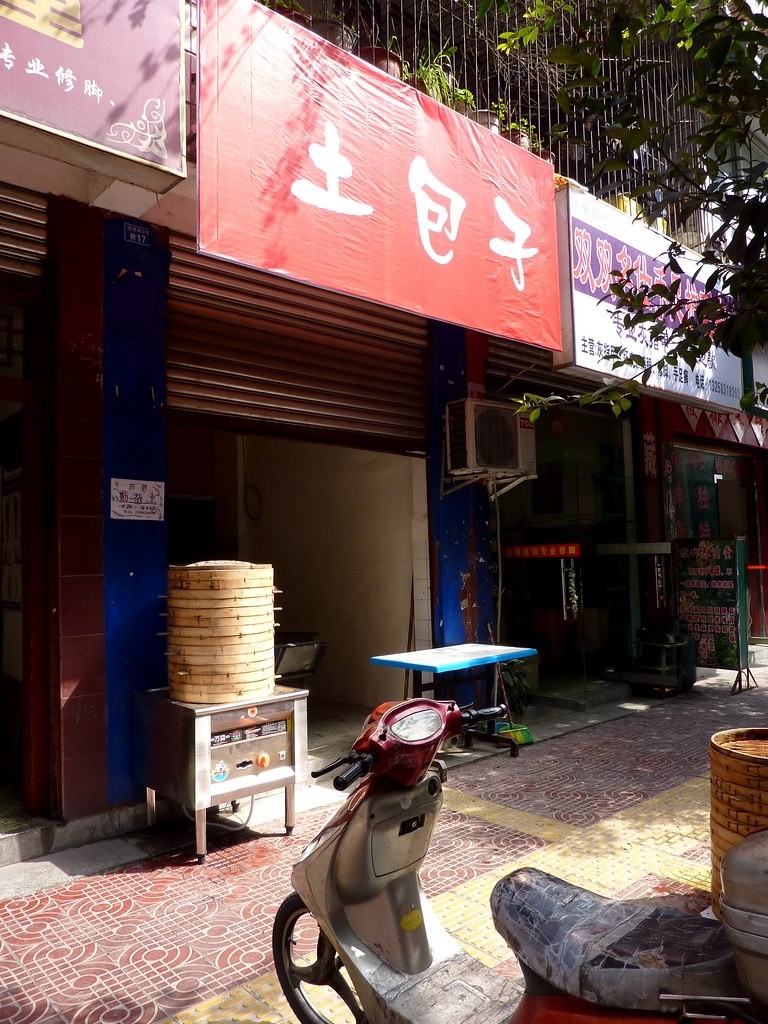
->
[273,688,756,1023]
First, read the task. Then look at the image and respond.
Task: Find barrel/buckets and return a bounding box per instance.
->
[519,656,539,691]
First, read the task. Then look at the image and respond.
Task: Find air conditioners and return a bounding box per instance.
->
[446,396,537,481]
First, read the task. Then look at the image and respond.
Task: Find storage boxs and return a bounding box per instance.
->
[602,195,668,236]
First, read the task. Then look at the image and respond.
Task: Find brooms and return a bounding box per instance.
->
[485,622,536,744]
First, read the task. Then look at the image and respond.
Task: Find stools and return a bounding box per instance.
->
[633,640,689,700]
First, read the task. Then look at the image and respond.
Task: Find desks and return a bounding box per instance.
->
[368,643,538,783]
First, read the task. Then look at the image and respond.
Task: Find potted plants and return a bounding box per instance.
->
[470,97,505,134]
[501,117,538,153]
[443,88,475,117]
[263,0,312,31]
[402,36,458,110]
[353,35,410,81]
[532,133,558,164]
[311,10,360,53]
